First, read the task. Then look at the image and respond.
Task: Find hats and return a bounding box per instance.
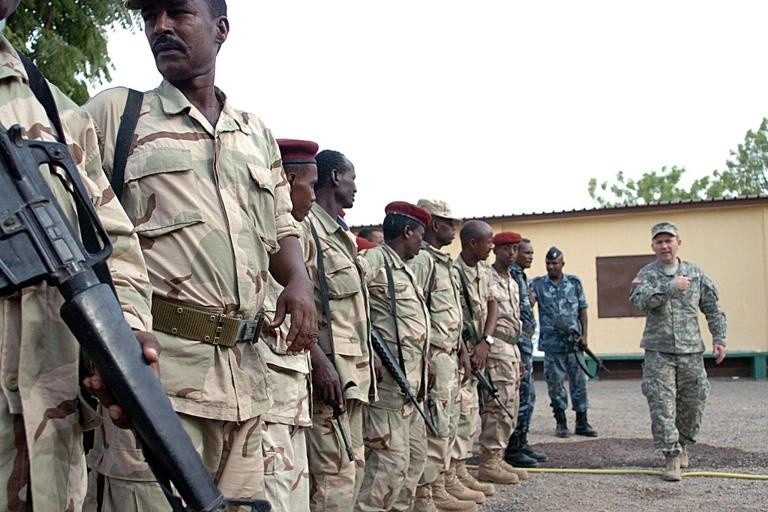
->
[546,246,562,259]
[356,236,376,251]
[418,199,461,223]
[651,222,677,239]
[385,201,432,227]
[276,138,319,164]
[493,232,521,245]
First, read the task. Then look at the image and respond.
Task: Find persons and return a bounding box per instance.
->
[1,1,319,512]
[274,139,548,512]
[626,220,730,483]
[528,247,599,437]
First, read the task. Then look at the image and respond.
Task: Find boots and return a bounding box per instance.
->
[663,445,688,481]
[554,411,569,437]
[575,411,598,436]
[433,444,548,511]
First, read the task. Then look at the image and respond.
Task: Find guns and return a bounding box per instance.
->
[556,324,610,376]
[371,318,443,438]
[462,330,514,421]
[1,121,270,512]
[329,401,356,461]
